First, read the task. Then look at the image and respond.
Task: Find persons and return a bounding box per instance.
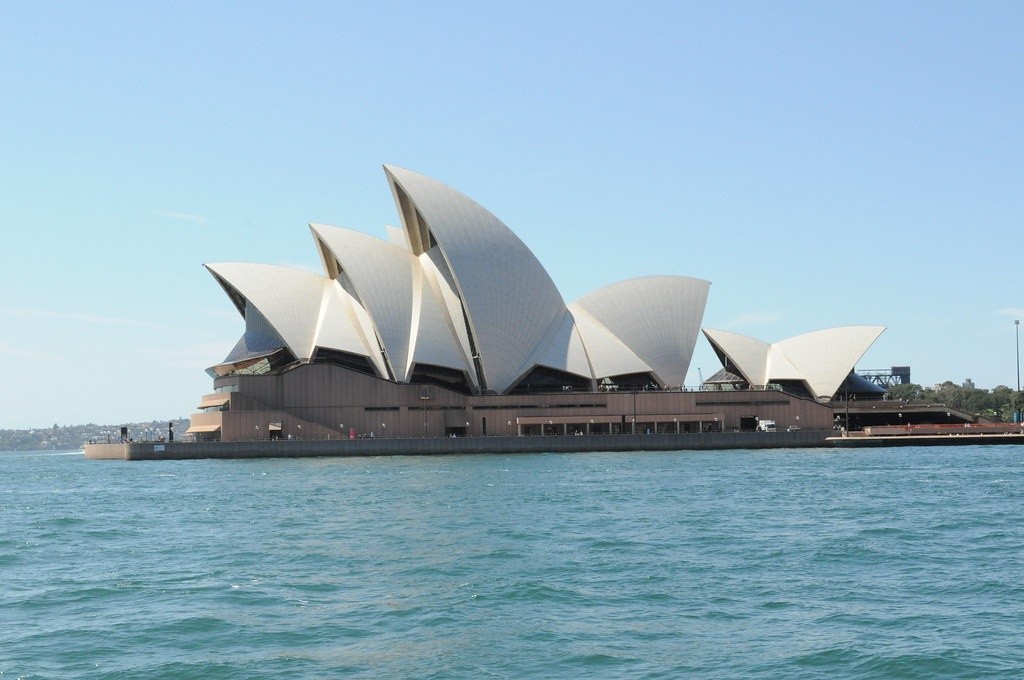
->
[833,394,1024,434]
[562,381,783,390]
[272,427,374,440]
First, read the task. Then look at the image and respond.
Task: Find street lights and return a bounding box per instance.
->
[1014,319,1020,391]
